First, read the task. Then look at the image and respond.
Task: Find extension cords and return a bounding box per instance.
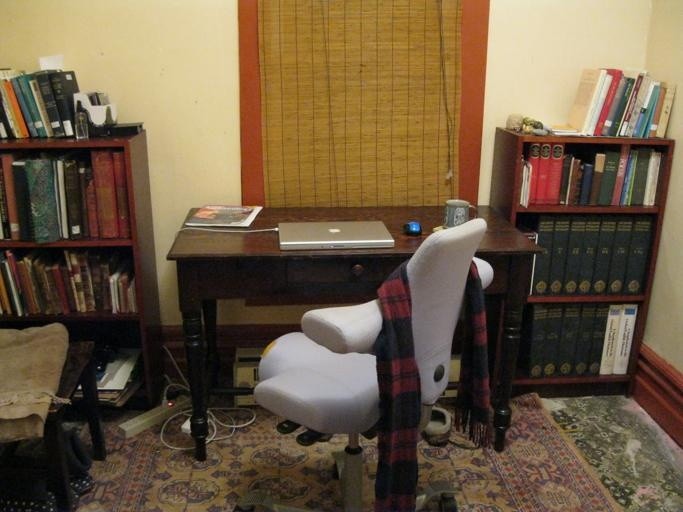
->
[118,394,191,440]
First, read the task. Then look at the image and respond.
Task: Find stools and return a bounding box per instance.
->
[0,338,107,512]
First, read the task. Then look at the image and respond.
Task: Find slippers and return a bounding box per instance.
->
[275,416,336,448]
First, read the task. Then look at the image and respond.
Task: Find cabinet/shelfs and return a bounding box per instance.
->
[489,124,675,401]
[0,122,168,412]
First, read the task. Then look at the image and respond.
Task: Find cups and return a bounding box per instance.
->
[443,199,479,230]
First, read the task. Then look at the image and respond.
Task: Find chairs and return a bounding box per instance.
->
[248,218,489,511]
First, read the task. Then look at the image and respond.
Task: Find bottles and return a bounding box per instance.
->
[75,112,89,140]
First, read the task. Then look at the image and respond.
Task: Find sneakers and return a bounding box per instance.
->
[420,401,453,448]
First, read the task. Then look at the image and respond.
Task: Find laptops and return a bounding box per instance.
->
[278,220,395,251]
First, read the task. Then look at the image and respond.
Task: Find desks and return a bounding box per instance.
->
[166,202,544,474]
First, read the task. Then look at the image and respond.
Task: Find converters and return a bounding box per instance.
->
[181,416,192,434]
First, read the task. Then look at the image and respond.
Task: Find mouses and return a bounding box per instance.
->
[404,222,422,236]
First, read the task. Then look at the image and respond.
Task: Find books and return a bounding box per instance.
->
[184,204,263,229]
[1,151,132,240]
[72,347,142,405]
[519,143,664,208]
[0,251,139,316]
[531,214,653,297]
[582,68,676,140]
[1,70,79,139]
[528,303,638,375]
